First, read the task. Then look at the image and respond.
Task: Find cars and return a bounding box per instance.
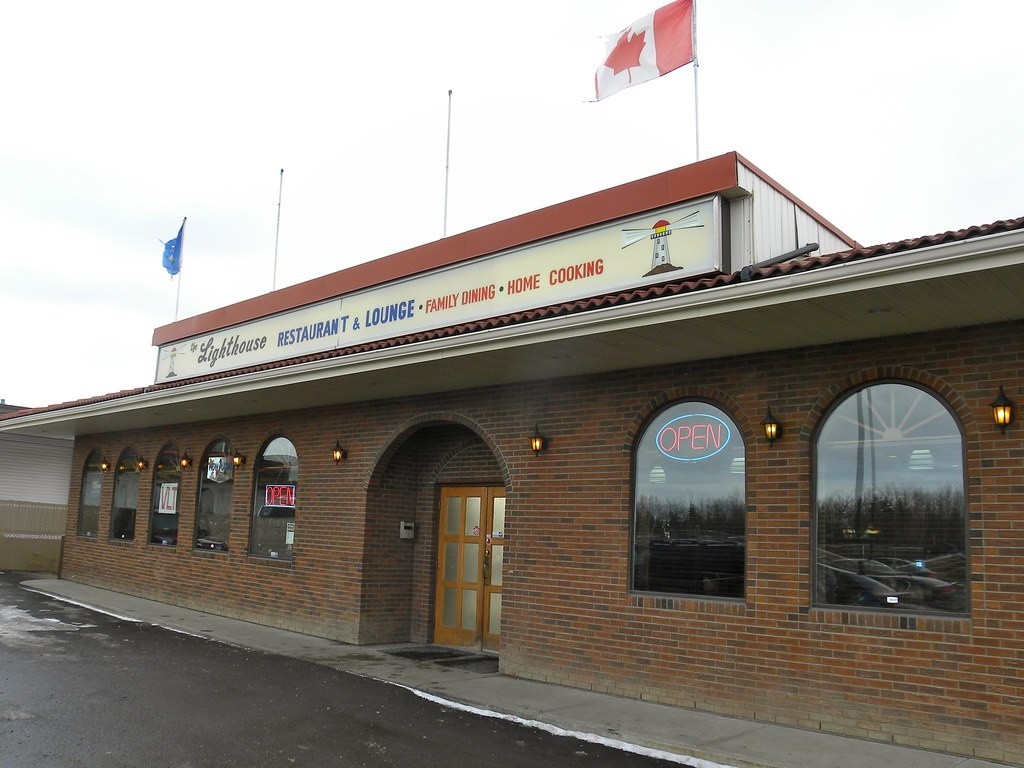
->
[634,537,746,597]
[817,546,965,612]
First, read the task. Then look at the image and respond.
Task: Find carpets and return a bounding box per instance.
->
[377,645,476,662]
[432,654,498,674]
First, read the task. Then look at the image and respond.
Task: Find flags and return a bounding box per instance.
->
[588,0,693,102]
[162,220,185,276]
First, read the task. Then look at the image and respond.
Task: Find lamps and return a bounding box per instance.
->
[330,438,348,466]
[232,448,246,470]
[759,405,783,448]
[100,456,110,473]
[137,454,149,472]
[180,450,193,471]
[528,423,548,458]
[988,385,1017,435]
